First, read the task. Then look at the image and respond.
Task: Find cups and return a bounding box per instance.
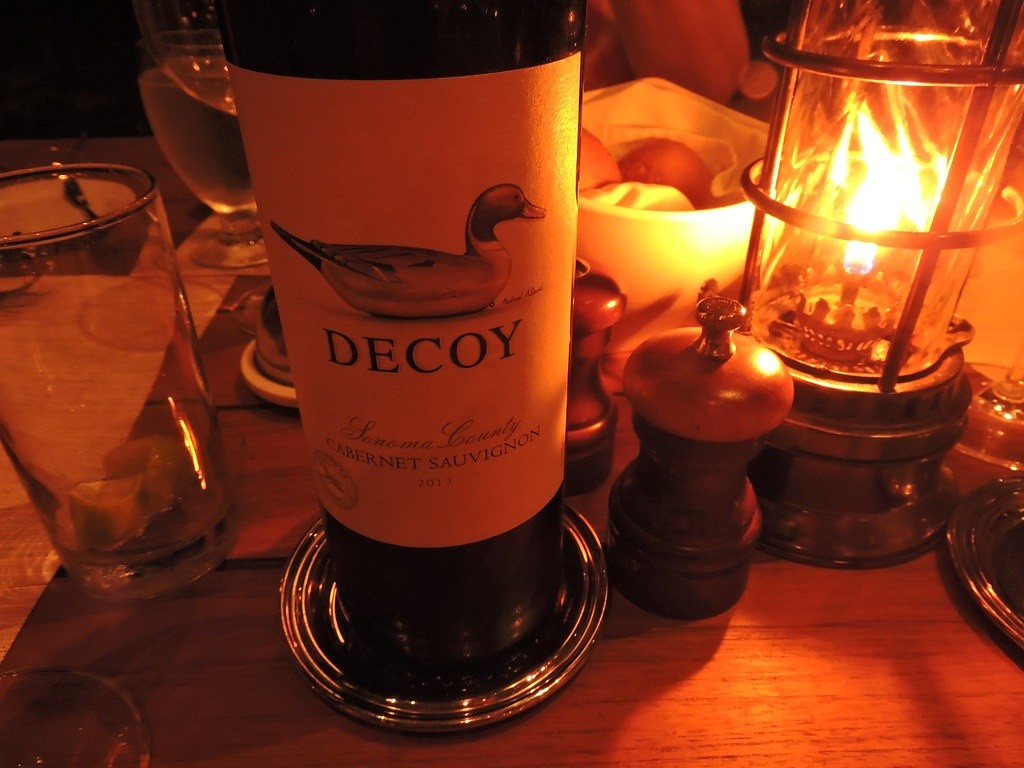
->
[0,164,240,601]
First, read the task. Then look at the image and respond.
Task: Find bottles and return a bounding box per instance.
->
[214,0,582,667]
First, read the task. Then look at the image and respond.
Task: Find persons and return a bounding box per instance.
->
[583,0,751,125]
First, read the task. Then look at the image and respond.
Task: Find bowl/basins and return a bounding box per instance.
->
[578,77,769,312]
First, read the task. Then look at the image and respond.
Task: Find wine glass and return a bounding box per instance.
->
[139,32,270,265]
[135,0,271,335]
[954,352,1024,470]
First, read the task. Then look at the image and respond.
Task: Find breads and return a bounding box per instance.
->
[577,129,713,208]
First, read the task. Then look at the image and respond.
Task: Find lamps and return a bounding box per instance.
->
[738,0,1024,571]
